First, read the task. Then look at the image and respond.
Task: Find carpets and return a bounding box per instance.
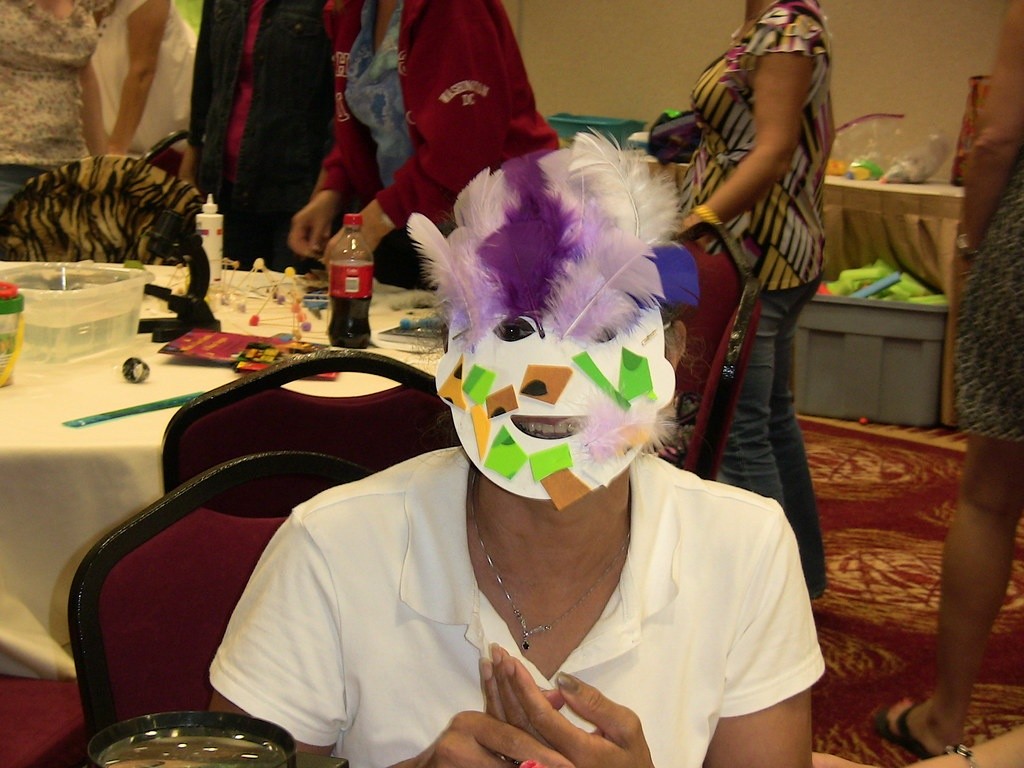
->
[794,417,1024,766]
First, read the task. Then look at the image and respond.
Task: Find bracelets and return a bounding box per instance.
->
[944,744,976,768]
[381,214,395,227]
[695,206,720,225]
[955,233,981,262]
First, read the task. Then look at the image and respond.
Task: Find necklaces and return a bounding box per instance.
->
[744,0,780,32]
[470,473,629,651]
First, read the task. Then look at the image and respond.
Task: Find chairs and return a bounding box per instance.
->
[0,154,206,267]
[0,673,92,767]
[159,348,462,499]
[67,450,375,737]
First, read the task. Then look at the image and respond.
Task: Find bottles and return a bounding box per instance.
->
[325,213,375,350]
[194,193,224,287]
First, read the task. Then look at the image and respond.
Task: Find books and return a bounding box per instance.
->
[375,326,442,348]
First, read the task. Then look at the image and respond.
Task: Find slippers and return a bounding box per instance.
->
[871,696,960,759]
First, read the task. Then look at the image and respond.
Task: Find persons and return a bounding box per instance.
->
[813,726,1024,768]
[0,0,106,207]
[680,0,836,613]
[288,0,561,267]
[180,0,333,272]
[211,135,824,768]
[872,0,1024,757]
[92,0,197,157]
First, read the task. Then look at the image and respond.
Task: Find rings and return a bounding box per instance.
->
[514,761,519,765]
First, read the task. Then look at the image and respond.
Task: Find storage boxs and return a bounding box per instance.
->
[548,111,650,150]
[0,260,158,365]
[792,294,949,429]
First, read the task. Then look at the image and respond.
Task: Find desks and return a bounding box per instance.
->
[0,259,446,674]
